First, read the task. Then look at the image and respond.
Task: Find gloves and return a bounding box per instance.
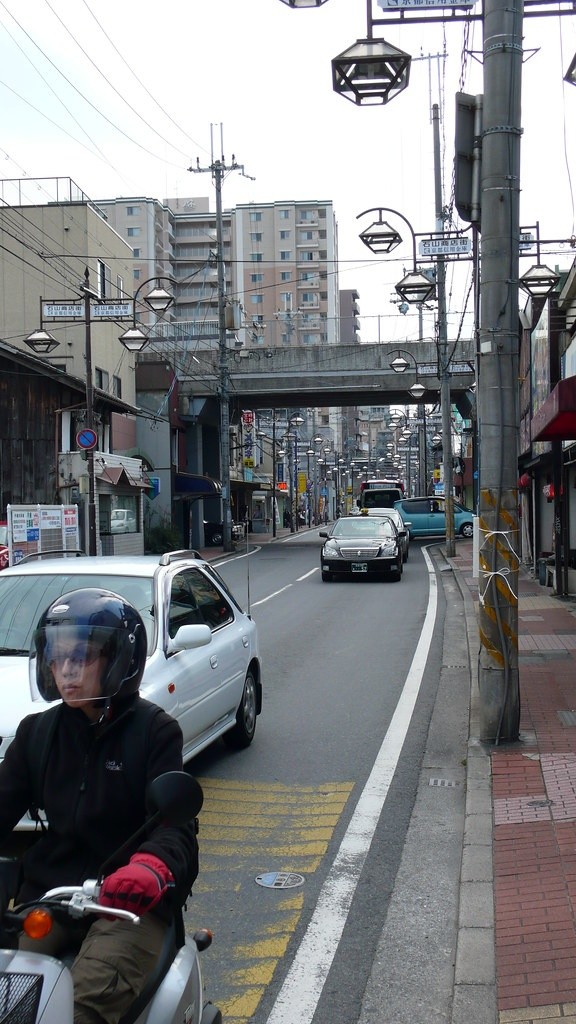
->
[97,851,175,922]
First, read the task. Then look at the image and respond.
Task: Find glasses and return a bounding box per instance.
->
[45,642,110,667]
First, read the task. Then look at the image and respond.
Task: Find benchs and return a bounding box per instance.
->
[545,566,576,594]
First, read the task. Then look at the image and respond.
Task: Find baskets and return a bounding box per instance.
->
[0,972,44,1024]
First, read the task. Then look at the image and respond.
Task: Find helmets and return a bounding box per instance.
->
[29,587,147,703]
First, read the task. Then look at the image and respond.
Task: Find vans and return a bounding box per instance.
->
[357,489,404,508]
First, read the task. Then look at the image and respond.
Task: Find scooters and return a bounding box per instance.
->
[1,770,225,1024]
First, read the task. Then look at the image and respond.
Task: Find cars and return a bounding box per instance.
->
[92,509,139,536]
[357,508,413,564]
[319,509,406,583]
[393,496,477,541]
[175,516,235,547]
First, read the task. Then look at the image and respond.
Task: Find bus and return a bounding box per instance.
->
[359,478,406,497]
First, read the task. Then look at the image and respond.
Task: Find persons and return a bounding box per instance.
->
[0,587,199,1024]
[254,505,341,531]
[432,501,443,512]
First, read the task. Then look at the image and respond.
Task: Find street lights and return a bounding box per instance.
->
[255,406,306,538]
[352,208,457,558]
[332,0,576,743]
[388,409,411,499]
[316,445,356,526]
[23,264,181,555]
[305,433,330,530]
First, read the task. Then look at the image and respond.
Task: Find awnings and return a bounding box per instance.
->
[530,376,576,442]
[96,466,151,488]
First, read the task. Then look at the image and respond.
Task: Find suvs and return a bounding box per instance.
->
[1,548,264,835]
